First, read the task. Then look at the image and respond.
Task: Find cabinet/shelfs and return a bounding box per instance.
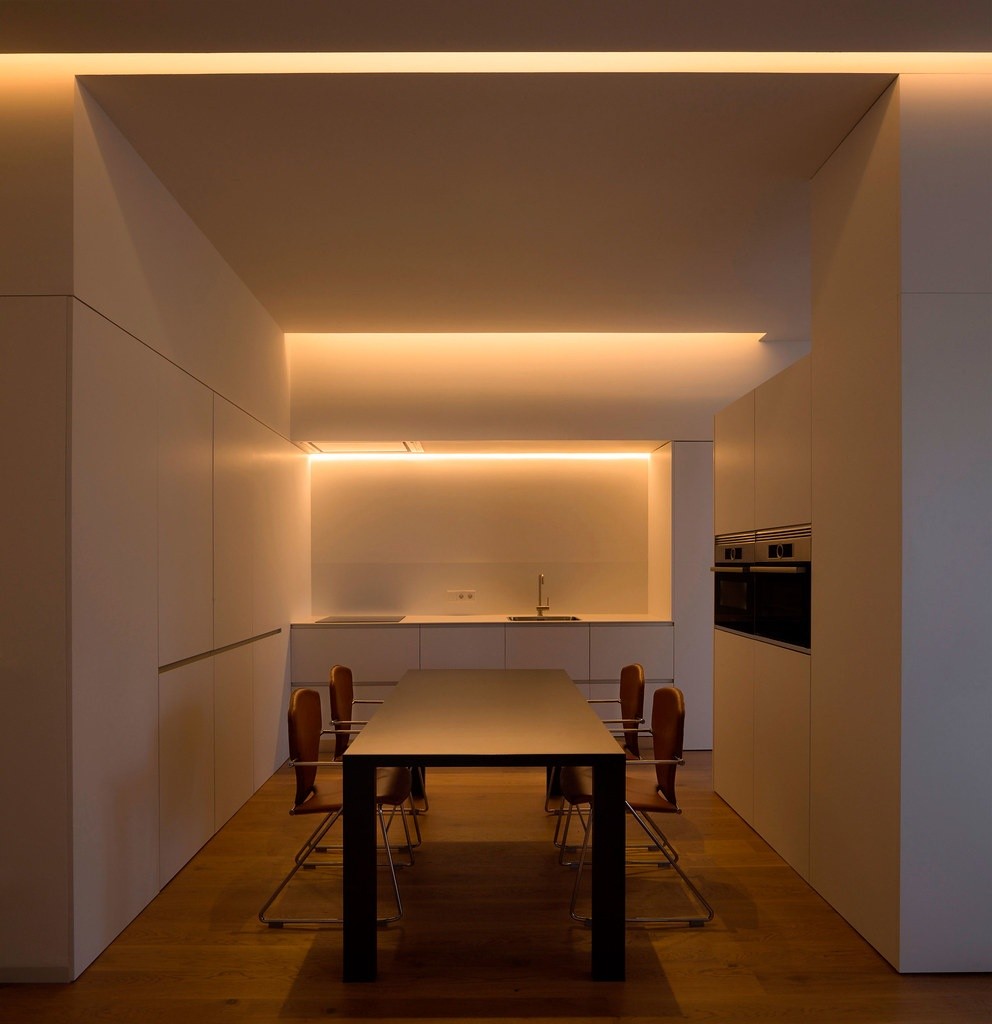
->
[289,624,419,681]
[290,682,399,741]
[590,622,674,679]
[419,623,506,669]
[158,354,283,892]
[569,680,589,704]
[714,629,811,888]
[505,622,590,680]
[589,680,674,737]
[712,352,811,536]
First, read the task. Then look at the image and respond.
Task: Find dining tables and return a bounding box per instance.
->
[342,667,626,981]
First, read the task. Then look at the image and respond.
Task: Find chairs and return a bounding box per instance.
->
[308,664,428,852]
[259,688,414,930]
[545,662,666,852]
[558,686,714,928]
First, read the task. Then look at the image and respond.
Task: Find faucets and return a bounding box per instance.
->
[536,573,550,616]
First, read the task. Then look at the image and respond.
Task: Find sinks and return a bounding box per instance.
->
[504,615,581,621]
